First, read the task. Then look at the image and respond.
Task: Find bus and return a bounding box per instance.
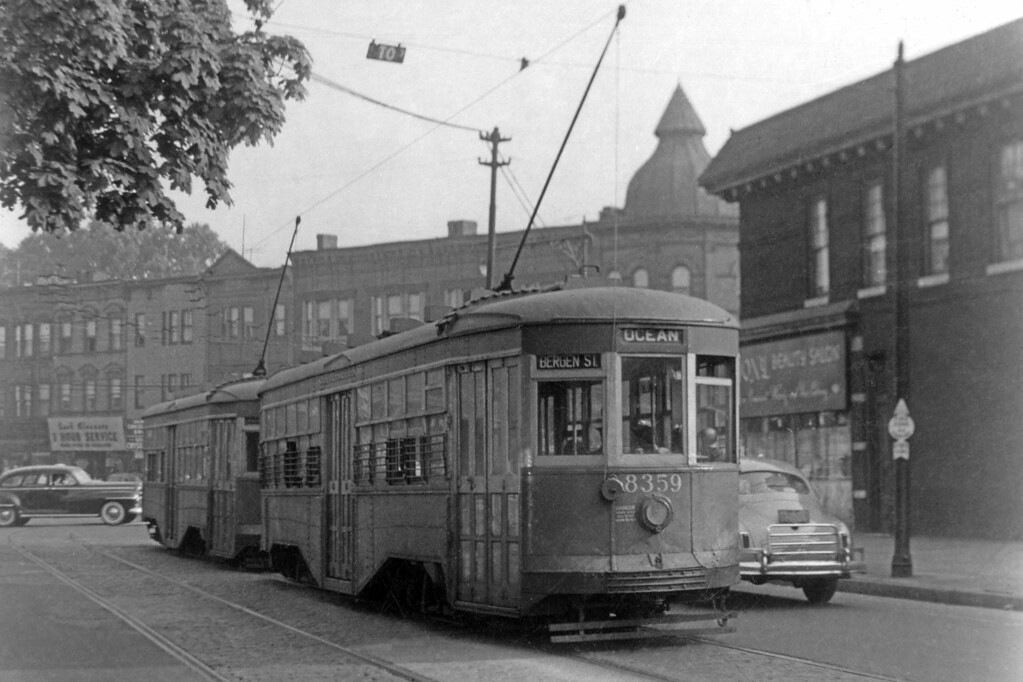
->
[139,285,743,645]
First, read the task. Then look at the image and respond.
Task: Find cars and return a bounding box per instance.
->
[741,458,864,602]
[0,463,137,527]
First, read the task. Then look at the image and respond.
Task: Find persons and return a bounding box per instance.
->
[51,473,62,485]
